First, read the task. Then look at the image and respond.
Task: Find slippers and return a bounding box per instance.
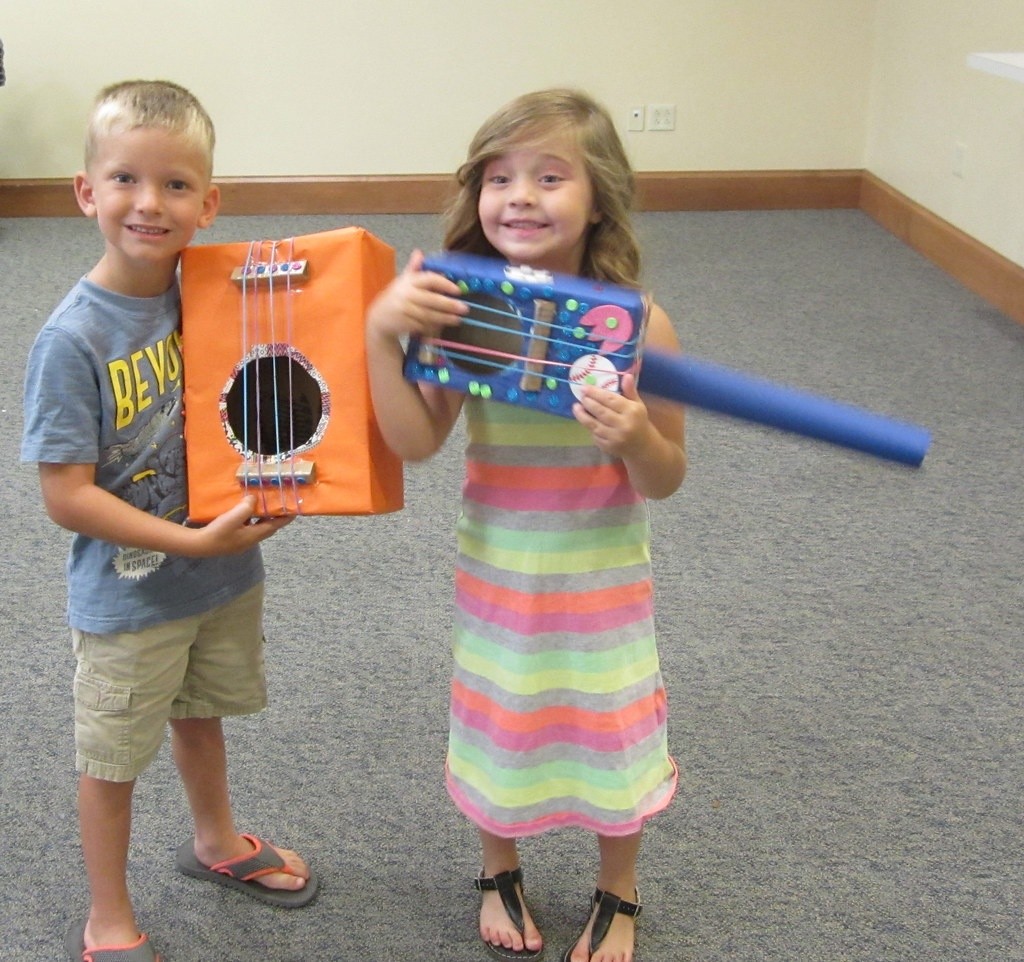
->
[175,831,318,907]
[65,917,169,962]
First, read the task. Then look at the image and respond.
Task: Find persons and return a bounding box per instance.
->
[19,80,312,962]
[364,88,683,962]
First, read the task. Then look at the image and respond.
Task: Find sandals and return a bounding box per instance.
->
[563,885,642,962]
[476,862,544,962]
